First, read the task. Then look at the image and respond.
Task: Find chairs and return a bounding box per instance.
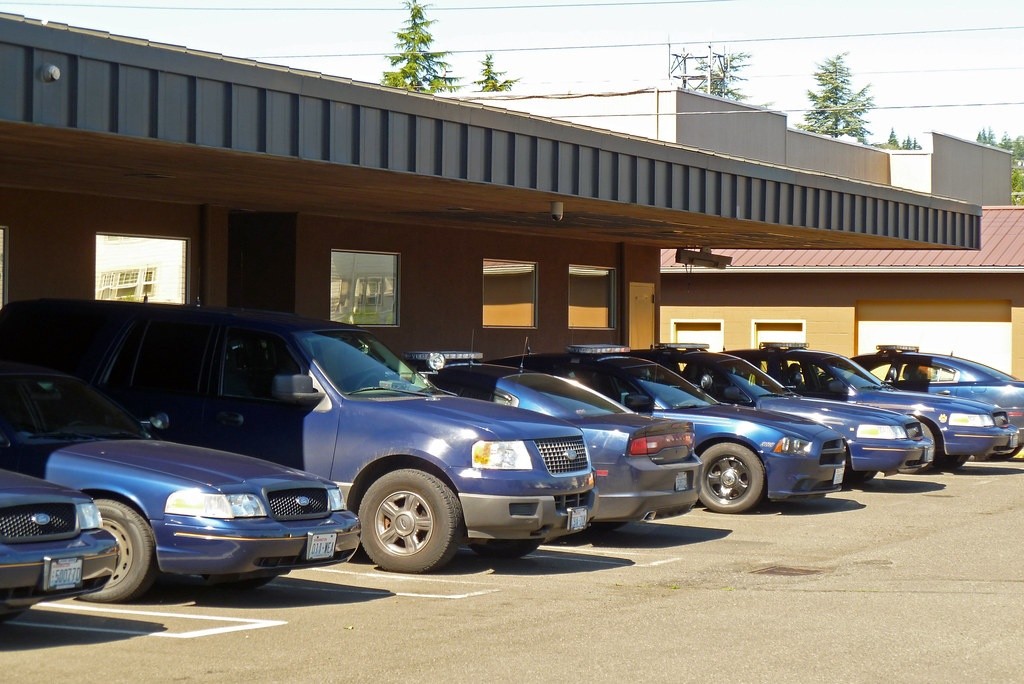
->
[903,363,918,380]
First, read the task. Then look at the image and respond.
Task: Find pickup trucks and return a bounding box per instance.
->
[0,297,600,578]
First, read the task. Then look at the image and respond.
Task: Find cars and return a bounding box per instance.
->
[420,363,701,535]
[789,349,1018,472]
[618,349,935,489]
[856,351,1024,460]
[0,462,120,635]
[483,350,850,514]
[1,356,365,603]
